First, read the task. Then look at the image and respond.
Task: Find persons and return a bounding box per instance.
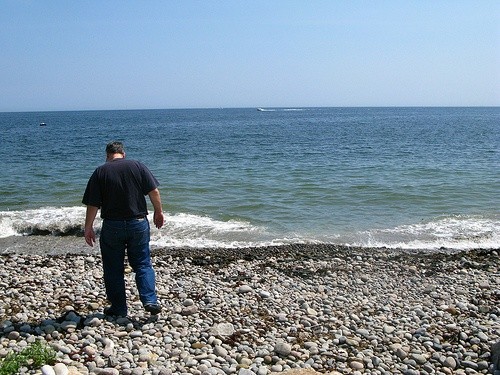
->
[82,141,164,317]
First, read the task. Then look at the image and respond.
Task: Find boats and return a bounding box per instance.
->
[40,121,47,126]
[256,106,264,112]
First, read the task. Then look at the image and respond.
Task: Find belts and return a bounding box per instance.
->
[104,215,144,222]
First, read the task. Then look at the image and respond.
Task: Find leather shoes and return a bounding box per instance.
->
[104,306,127,318]
[146,304,162,315]
